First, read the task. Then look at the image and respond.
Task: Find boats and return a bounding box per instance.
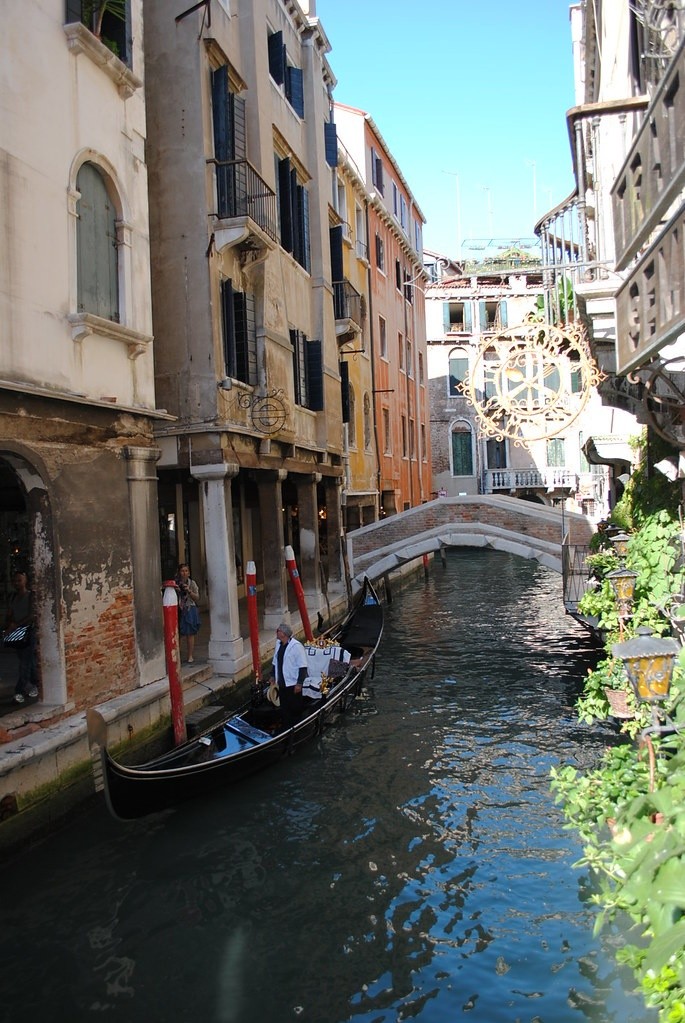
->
[85,571,385,821]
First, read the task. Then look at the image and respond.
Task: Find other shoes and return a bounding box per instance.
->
[188,659,193,662]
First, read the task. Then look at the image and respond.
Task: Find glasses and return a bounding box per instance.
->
[276,628,281,632]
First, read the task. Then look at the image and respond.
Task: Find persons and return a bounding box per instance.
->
[4,571,39,705]
[235,553,243,585]
[174,564,200,664]
[269,622,308,734]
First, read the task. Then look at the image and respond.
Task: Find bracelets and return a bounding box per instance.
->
[238,576,242,577]
[188,591,193,595]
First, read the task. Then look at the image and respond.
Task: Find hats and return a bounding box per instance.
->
[266,686,280,707]
